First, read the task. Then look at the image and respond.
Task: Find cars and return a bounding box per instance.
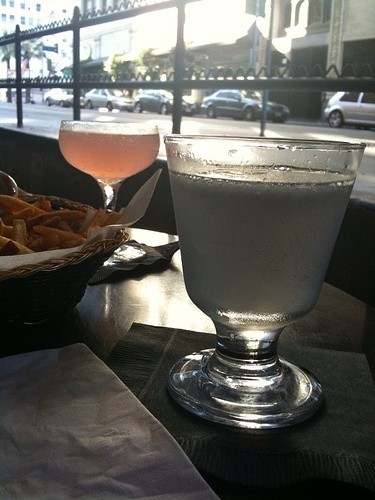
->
[129,89,199,117]
[43,88,85,108]
[200,88,290,124]
[323,91,375,130]
[84,87,135,113]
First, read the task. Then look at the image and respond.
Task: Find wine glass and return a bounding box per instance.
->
[163,136,366,430]
[59,120,160,263]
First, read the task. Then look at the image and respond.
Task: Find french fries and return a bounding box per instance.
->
[0,193,120,256]
